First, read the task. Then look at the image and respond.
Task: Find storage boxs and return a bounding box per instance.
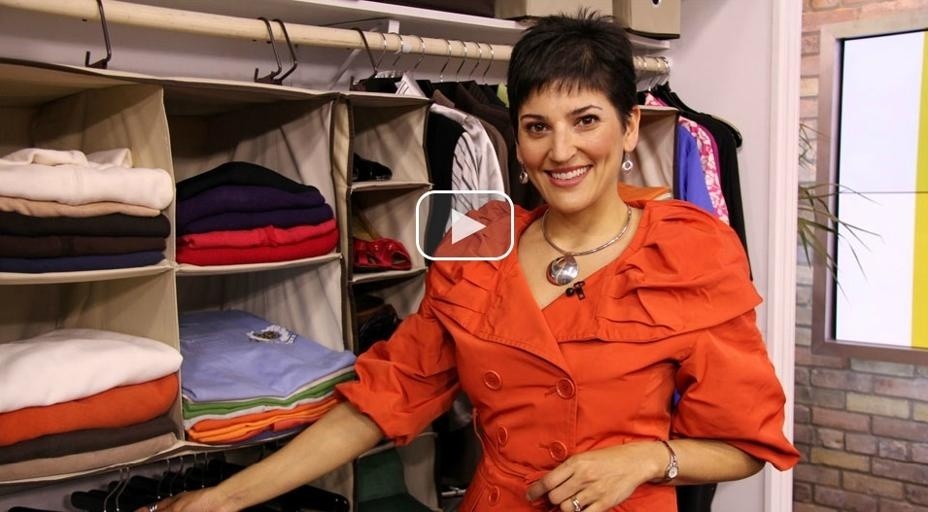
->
[493,0,681,40]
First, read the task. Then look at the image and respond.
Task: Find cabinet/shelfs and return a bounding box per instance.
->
[0,57,439,512]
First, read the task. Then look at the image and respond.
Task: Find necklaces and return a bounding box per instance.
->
[542,207,632,286]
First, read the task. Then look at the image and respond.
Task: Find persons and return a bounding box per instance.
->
[131,6,804,512]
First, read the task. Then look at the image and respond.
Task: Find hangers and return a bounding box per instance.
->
[357,33,673,91]
[101,437,277,512]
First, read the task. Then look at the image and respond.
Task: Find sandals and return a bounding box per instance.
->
[352,206,411,270]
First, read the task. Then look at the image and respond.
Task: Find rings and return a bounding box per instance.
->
[571,498,581,511]
[147,501,159,512]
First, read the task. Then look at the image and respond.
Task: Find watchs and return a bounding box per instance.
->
[657,437,678,482]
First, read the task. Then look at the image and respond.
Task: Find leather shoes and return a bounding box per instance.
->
[354,151,391,183]
